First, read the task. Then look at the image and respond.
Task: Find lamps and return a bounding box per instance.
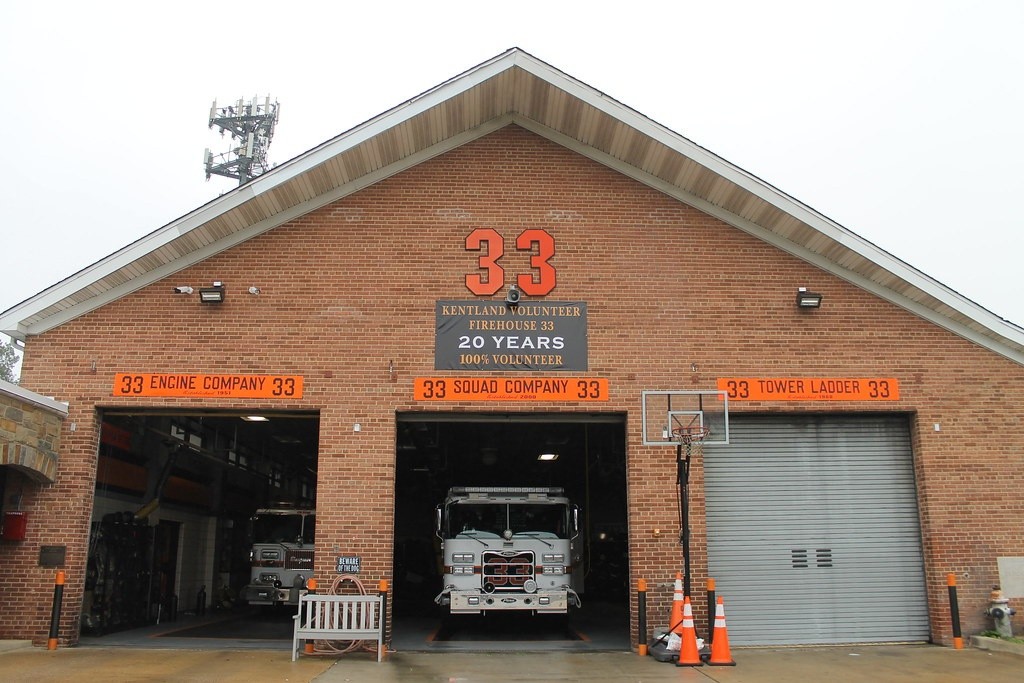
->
[198,286,225,303]
[797,293,821,307]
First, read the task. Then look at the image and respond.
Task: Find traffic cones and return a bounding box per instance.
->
[700,596,736,667]
[673,594,706,667]
[668,571,686,637]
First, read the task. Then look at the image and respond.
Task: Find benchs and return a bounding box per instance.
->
[290,590,384,663]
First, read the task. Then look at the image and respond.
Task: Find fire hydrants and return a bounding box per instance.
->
[983,584,1017,639]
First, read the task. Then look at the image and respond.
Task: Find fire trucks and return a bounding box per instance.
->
[246,502,316,611]
[435,486,585,628]
